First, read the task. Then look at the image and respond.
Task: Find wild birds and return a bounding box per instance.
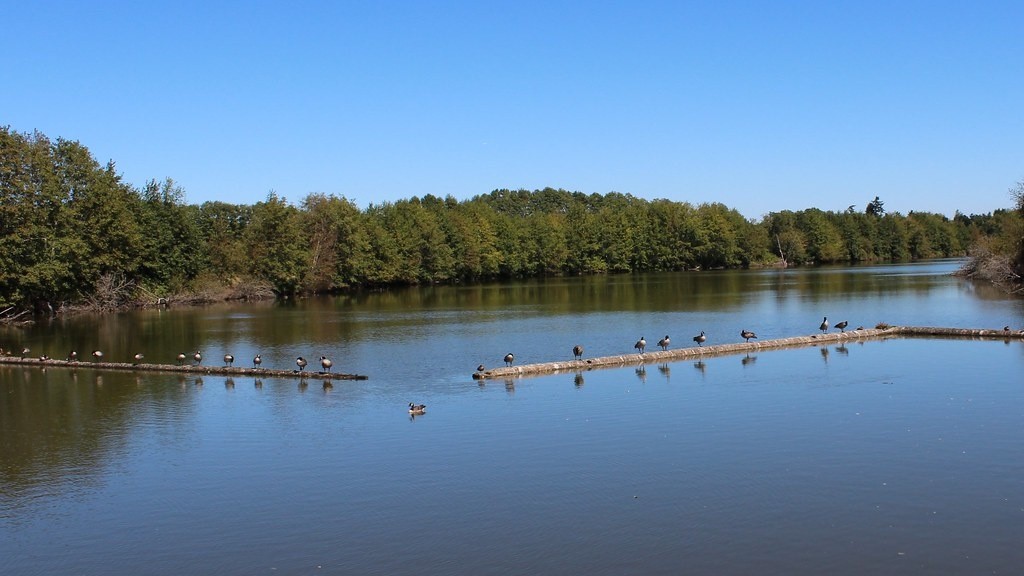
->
[741,330,758,343]
[176,350,202,366]
[693,331,706,347]
[477,365,485,373]
[296,356,334,373]
[128,351,144,364]
[573,345,583,360]
[634,335,670,354]
[819,317,864,334]
[504,353,514,367]
[223,353,262,369]
[0,347,105,363]
[408,402,426,415]
[1003,325,1009,331]
